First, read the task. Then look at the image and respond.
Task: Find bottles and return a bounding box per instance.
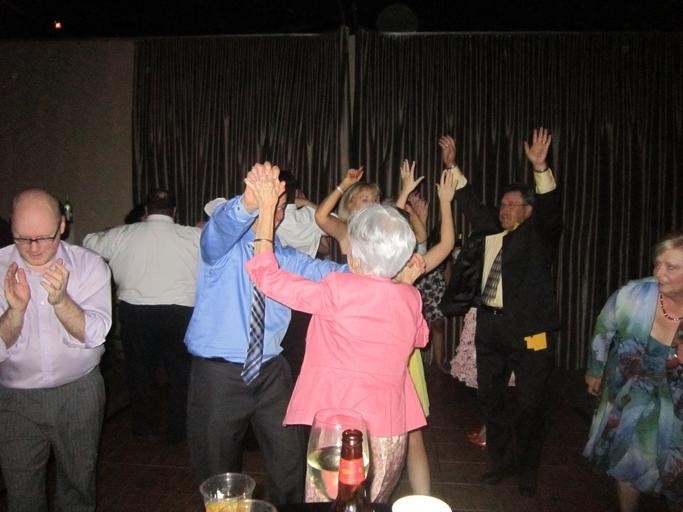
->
[331,431,376,511]
[63,195,72,222]
[451,232,463,264]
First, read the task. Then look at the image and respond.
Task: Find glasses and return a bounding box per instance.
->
[11,228,58,246]
[503,201,526,209]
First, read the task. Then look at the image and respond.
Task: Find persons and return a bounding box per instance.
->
[1,189,202,510]
[183,127,562,512]
[581,237,683,512]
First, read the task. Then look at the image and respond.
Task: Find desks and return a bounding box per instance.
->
[274,502,392,512]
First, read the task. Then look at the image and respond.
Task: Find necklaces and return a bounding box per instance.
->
[661,290,683,321]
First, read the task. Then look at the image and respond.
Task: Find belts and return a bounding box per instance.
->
[484,306,505,320]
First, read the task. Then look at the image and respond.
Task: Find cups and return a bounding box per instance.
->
[216,498,276,512]
[390,494,453,512]
[198,472,256,511]
[307,408,371,501]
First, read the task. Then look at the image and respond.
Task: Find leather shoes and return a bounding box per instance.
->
[483,468,515,483]
[469,432,486,445]
[520,471,536,496]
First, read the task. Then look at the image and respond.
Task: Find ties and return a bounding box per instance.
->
[242,278,265,386]
[481,244,503,305]
